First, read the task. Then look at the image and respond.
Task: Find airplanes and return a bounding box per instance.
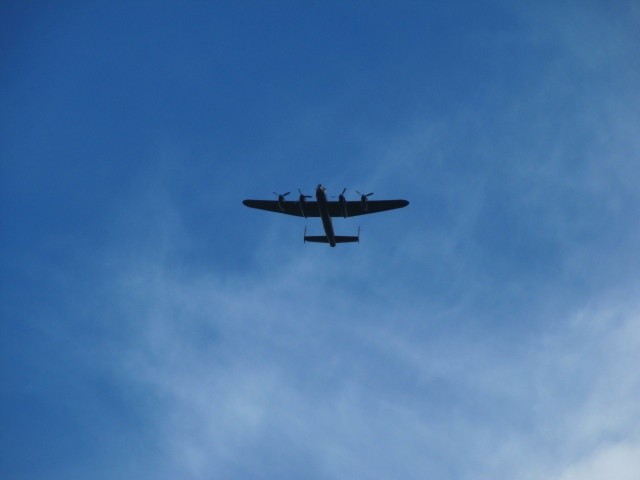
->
[243,184,409,246]
